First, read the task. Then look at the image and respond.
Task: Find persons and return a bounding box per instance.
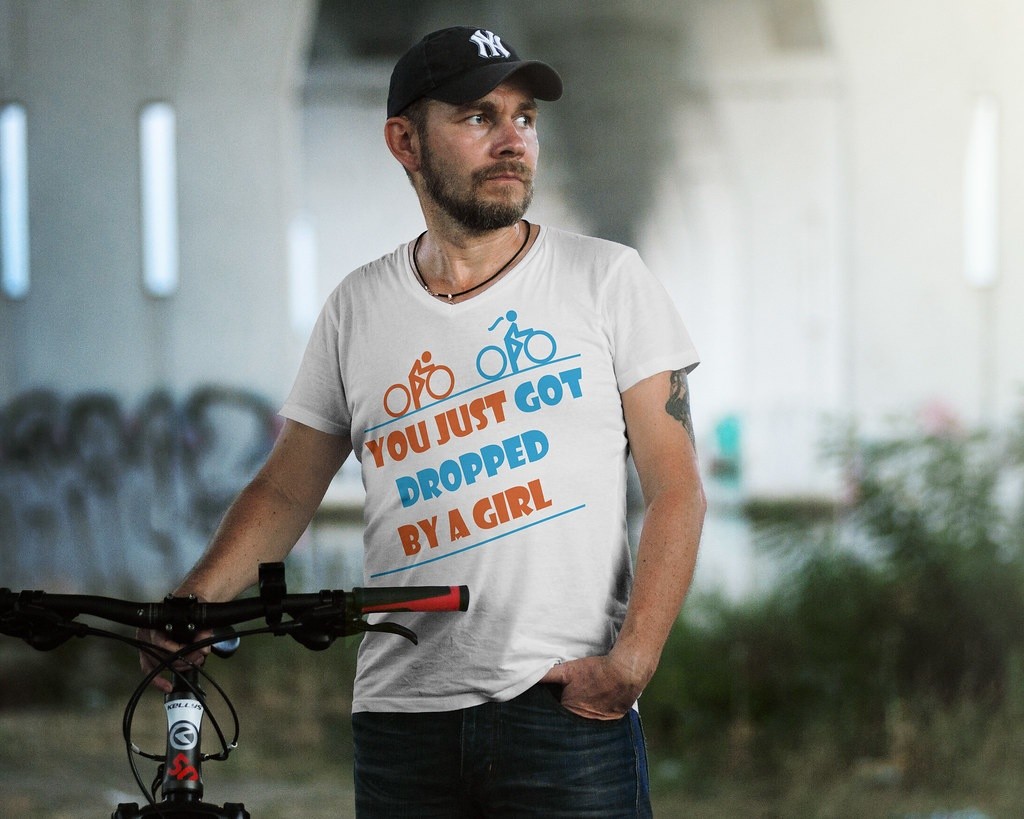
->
[140,25,713,819]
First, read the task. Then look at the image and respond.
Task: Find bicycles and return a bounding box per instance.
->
[1,560,473,818]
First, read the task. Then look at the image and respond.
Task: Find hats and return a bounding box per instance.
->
[384,25,570,124]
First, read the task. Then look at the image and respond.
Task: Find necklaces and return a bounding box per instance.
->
[409,207,533,305]
